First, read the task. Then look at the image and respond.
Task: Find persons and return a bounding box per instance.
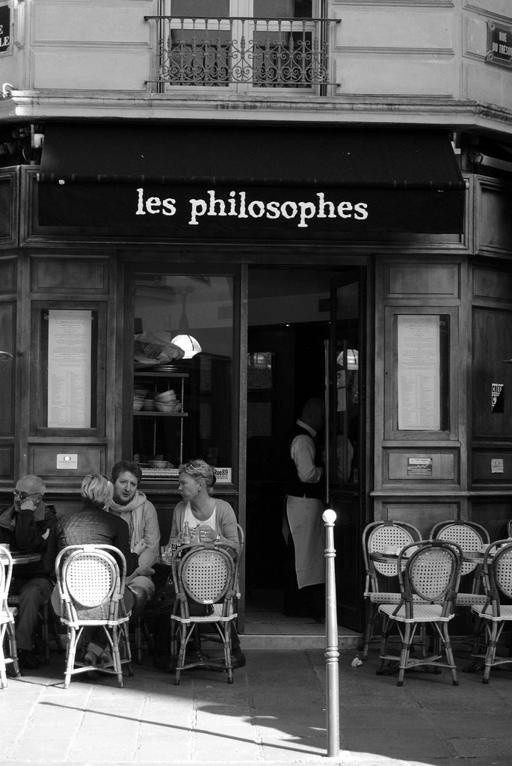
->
[281,398,325,617]
[140,458,246,670]
[102,460,160,610]
[0,474,59,676]
[41,473,147,676]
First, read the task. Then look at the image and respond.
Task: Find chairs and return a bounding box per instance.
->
[170,523,244,685]
[52,544,154,687]
[359,519,512,687]
[0,504,56,688]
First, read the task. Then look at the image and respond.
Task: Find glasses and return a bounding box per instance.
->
[13,489,40,500]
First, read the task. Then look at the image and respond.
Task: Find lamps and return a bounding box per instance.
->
[171,288,203,361]
[336,347,359,370]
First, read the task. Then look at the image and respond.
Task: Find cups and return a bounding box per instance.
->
[199,526,212,543]
[382,545,418,559]
[161,546,170,561]
[478,544,496,552]
[170,535,178,555]
[0,544,10,553]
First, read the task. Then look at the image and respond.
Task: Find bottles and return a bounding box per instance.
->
[181,521,192,556]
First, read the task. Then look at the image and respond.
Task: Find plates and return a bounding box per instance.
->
[155,364,178,373]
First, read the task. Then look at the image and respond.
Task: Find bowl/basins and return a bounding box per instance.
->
[149,460,168,468]
[133,390,178,412]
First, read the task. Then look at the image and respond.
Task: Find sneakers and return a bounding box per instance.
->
[85,651,113,679]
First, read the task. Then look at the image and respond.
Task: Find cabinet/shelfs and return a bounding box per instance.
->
[134,365,188,469]
